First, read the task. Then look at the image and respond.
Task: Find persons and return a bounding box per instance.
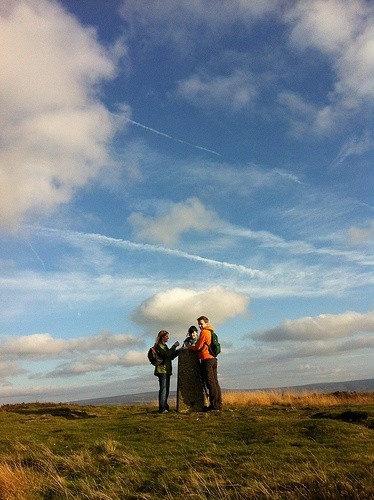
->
[147,316,222,413]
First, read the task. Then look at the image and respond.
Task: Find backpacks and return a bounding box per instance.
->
[204,329,221,356]
[148,346,164,365]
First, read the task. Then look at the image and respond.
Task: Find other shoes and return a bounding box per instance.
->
[160,404,169,413]
[203,404,222,412]
